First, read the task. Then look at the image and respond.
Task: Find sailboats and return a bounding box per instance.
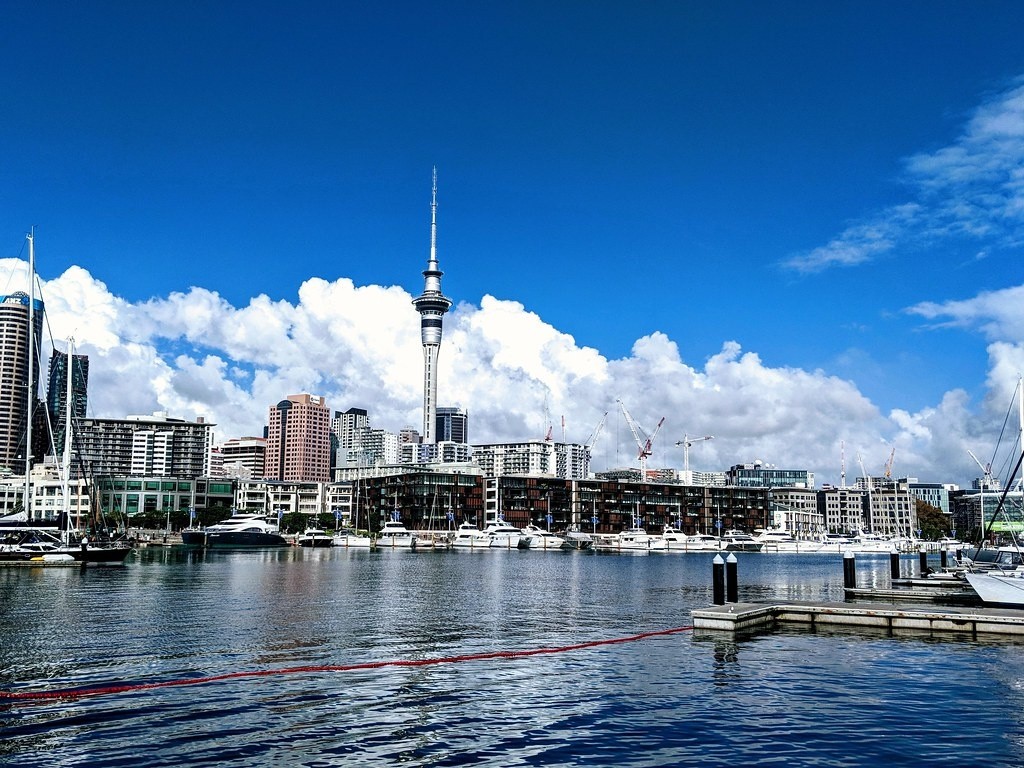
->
[964,369,1024,603]
[0,225,135,570]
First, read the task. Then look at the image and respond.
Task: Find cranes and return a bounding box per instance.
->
[840,440,906,490]
[543,398,716,482]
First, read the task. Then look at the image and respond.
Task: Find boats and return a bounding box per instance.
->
[521,476,974,553]
[298,525,334,547]
[330,461,457,549]
[181,513,292,547]
[455,518,540,549]
